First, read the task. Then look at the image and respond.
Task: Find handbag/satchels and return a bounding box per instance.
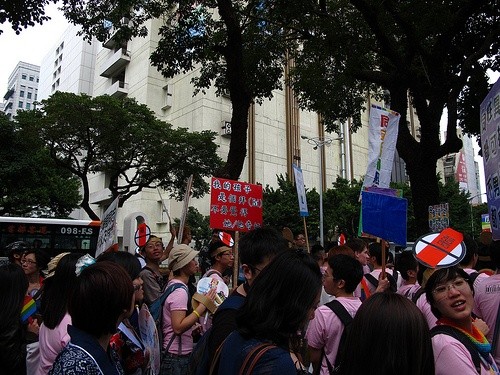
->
[26,341,40,375]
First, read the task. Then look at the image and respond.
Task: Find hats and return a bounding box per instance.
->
[211,245,232,257]
[5,241,36,252]
[168,244,200,271]
[43,252,71,280]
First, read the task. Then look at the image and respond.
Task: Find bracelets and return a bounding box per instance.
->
[193,310,201,318]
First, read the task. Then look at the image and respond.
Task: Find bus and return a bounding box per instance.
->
[0,216,118,272]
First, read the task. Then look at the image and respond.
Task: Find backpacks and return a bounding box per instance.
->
[149,283,190,352]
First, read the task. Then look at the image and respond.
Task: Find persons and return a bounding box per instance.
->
[207,246,323,375]
[0,223,500,375]
[412,228,500,375]
[46,259,136,375]
[334,290,435,375]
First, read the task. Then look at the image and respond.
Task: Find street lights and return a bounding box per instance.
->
[301,135,344,246]
[466,192,487,240]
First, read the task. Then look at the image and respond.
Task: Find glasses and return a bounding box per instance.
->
[323,273,337,279]
[295,238,304,241]
[432,278,468,296]
[21,260,36,264]
[188,233,193,236]
